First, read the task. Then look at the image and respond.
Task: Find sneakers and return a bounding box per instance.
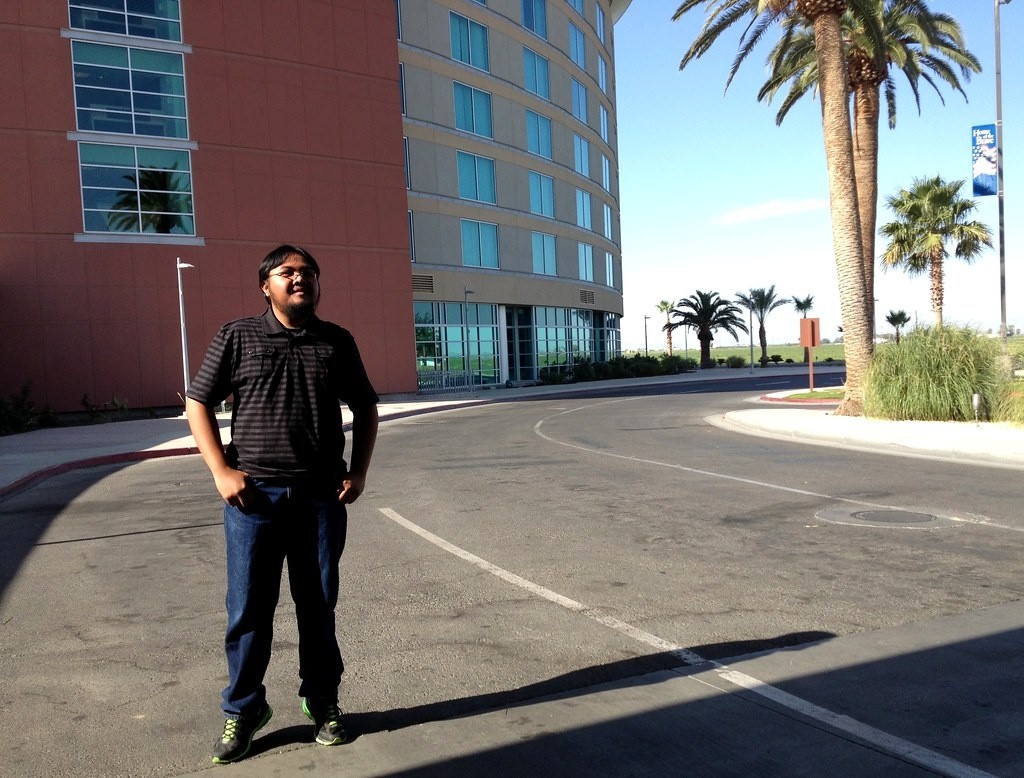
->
[301,690,347,745]
[209,699,273,763]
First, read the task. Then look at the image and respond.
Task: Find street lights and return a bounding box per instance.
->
[463,285,475,393]
[993,0,1014,338]
[176,256,196,416]
[749,293,759,374]
[644,315,651,357]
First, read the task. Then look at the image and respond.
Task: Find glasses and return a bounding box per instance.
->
[267,268,318,282]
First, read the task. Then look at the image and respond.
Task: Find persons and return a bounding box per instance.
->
[185,244,380,764]
